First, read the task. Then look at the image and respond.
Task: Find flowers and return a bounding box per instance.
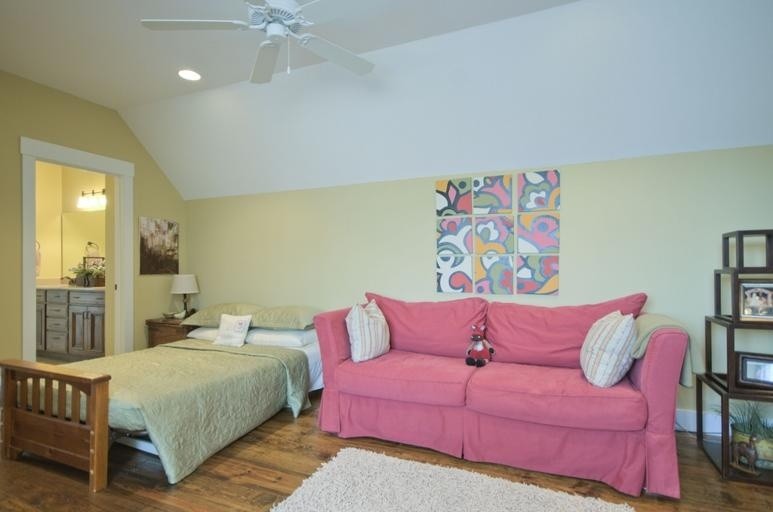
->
[68,259,105,277]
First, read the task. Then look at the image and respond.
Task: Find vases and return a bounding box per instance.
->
[74,272,103,287]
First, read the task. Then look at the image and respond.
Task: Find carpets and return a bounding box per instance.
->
[269,446,635,512]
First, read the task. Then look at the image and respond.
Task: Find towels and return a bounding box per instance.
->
[629,315,693,390]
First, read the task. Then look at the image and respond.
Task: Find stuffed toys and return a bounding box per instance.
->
[464,323,495,367]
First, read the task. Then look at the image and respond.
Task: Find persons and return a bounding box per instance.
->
[744,288,772,311]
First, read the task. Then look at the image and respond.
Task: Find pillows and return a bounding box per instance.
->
[579,310,640,389]
[343,298,391,363]
[180,302,320,348]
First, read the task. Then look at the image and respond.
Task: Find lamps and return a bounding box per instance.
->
[76,188,105,210]
[169,274,199,315]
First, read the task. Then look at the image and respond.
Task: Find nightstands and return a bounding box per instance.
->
[144,315,197,348]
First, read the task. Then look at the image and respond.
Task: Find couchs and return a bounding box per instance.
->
[312,293,689,499]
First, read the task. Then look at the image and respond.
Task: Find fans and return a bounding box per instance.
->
[140,1,376,84]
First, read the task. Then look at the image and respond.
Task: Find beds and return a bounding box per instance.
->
[0,298,325,492]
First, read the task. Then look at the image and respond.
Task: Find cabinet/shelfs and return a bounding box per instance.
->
[36,289,105,361]
[691,229,773,485]
